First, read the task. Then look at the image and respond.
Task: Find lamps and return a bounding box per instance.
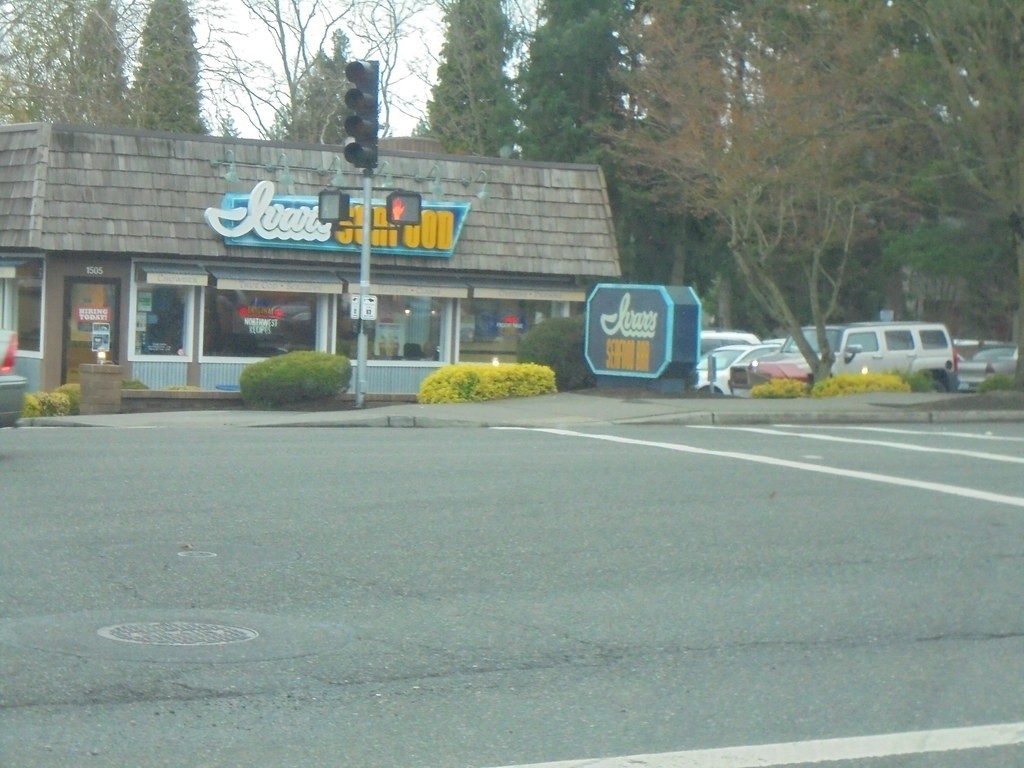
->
[97,347,106,364]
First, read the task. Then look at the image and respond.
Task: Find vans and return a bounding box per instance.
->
[727,322,960,398]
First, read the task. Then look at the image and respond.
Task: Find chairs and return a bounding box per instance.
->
[404,344,422,361]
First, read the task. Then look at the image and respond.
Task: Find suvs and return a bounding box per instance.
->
[700,328,761,356]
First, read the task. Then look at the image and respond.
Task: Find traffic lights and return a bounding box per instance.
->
[317,188,350,224]
[386,190,421,226]
[344,60,379,168]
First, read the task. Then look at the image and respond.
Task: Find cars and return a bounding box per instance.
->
[0,329,28,427]
[404,300,436,320]
[957,344,1018,391]
[695,344,797,396]
